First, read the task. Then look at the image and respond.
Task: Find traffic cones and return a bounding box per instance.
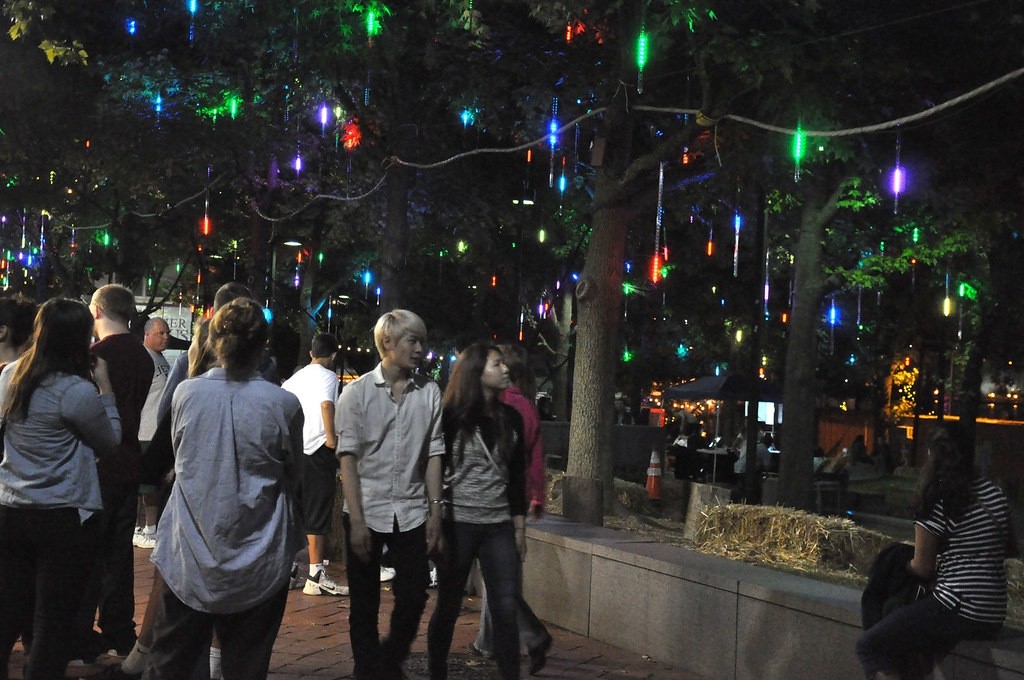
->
[643,449,666,502]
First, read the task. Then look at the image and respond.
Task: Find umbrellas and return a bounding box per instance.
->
[663,374,786,484]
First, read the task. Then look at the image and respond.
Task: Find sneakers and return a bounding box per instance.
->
[289,565,306,590]
[303,569,349,595]
[133,528,156,548]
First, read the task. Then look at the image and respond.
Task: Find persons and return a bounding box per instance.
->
[855,421,1008,680]
[280,333,350,596]
[0,283,304,680]
[615,399,634,425]
[723,417,771,485]
[426,343,553,680]
[336,309,445,680]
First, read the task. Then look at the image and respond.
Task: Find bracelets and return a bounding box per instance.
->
[428,498,443,502]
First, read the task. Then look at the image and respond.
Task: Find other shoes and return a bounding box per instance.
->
[79,663,142,680]
[380,567,396,581]
[529,635,553,675]
[466,643,482,654]
